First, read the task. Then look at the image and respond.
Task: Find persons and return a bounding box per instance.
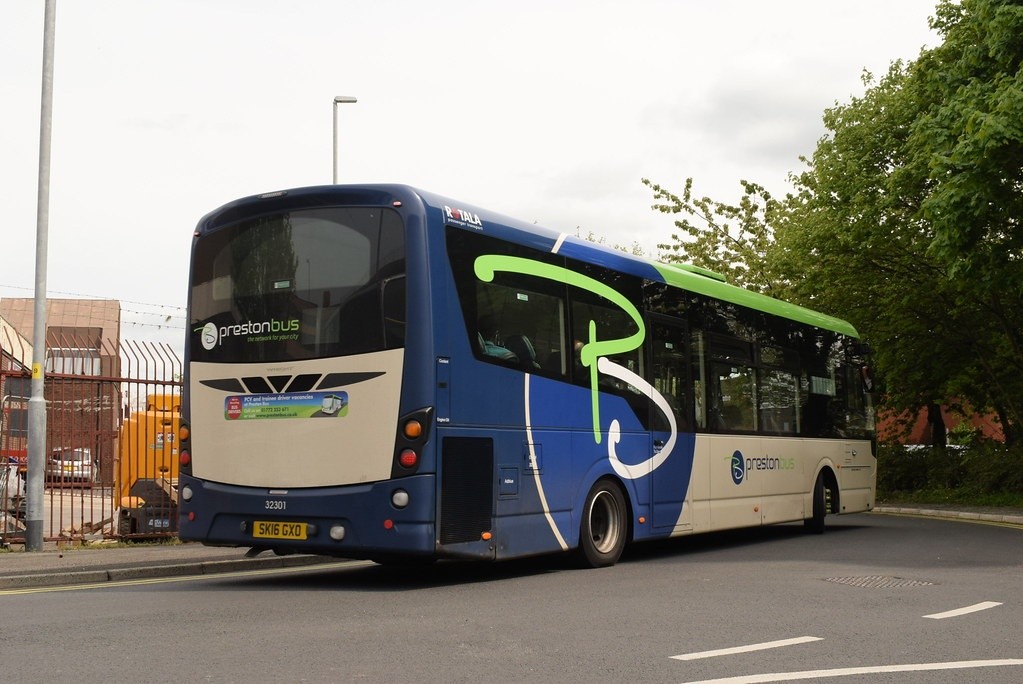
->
[479,313,585,381]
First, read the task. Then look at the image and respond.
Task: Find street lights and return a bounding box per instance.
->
[333,95,357,184]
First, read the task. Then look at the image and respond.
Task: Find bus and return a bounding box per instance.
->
[176,182,878,574]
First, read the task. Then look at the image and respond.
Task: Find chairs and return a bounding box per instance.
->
[660,392,789,435]
[474,325,620,387]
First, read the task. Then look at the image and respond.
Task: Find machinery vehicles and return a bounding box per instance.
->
[115,393,180,542]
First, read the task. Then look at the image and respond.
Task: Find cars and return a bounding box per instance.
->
[46,447,98,488]
